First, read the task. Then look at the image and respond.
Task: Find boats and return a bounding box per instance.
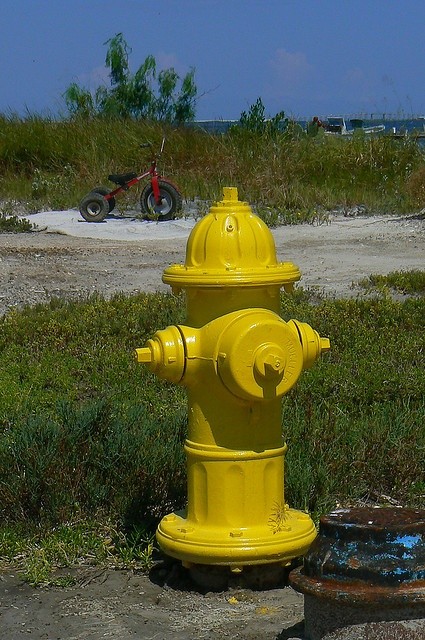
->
[323,117,386,136]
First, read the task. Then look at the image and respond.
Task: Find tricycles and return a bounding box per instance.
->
[79,137,182,222]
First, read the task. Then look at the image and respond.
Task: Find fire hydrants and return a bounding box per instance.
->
[134,187,332,588]
